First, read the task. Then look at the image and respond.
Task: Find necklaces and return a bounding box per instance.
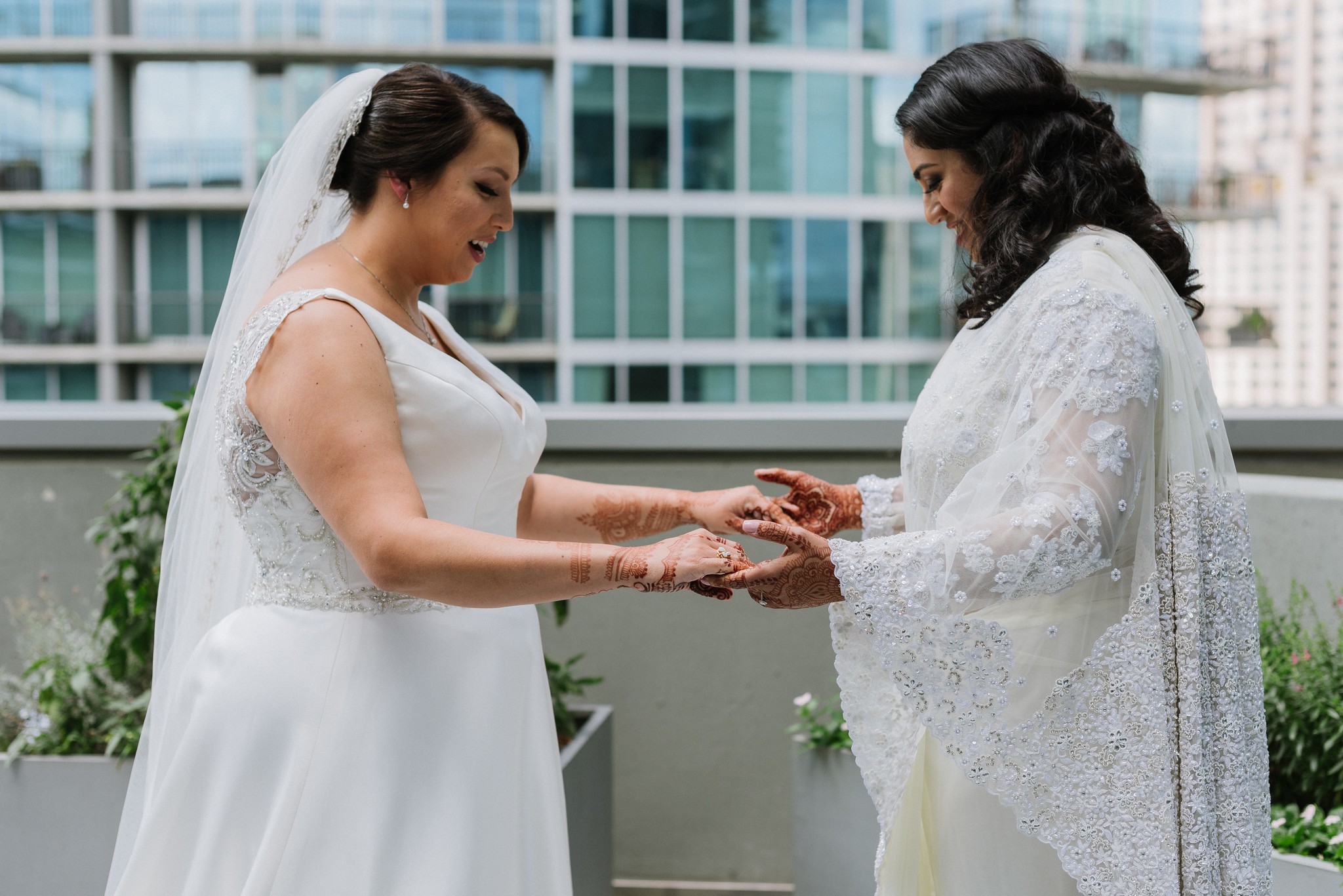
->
[334,236,438,347]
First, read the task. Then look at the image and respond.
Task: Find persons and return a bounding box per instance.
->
[96,57,807,896]
[697,38,1282,894]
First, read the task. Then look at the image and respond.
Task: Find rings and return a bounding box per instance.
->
[757,593,767,606]
[716,544,732,559]
[765,495,773,502]
